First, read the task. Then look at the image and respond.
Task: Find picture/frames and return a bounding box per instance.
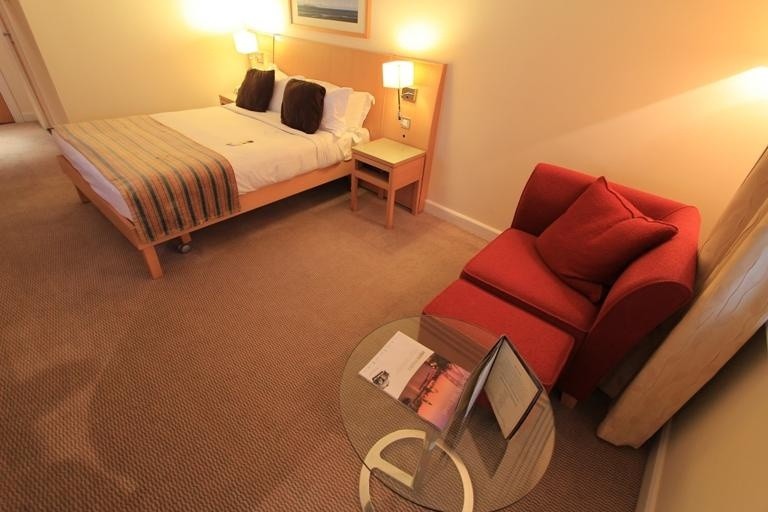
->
[287,0,371,39]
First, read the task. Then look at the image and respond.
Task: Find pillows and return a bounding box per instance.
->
[233,64,375,138]
[533,176,678,306]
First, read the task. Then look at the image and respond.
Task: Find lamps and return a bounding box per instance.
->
[381,60,414,129]
[233,31,257,71]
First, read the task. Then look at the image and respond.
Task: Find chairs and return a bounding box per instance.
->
[460,162,700,410]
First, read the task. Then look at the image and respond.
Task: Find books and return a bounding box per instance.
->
[358,330,472,433]
[442,391,508,479]
[455,333,544,441]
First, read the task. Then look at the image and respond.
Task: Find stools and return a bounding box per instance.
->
[418,276,575,418]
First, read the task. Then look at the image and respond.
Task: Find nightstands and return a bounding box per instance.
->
[350,137,425,226]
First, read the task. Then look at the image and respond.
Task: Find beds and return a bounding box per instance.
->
[50,33,447,280]
